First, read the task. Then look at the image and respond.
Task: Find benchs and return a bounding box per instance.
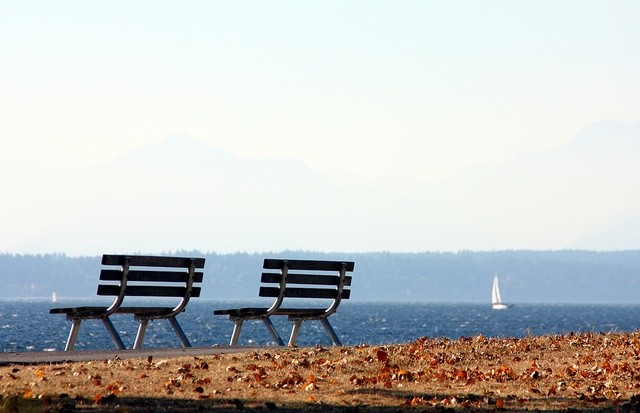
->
[47,255,207,354]
[214,259,355,348]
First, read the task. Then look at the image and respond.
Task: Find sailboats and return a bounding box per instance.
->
[490,272,510,310]
